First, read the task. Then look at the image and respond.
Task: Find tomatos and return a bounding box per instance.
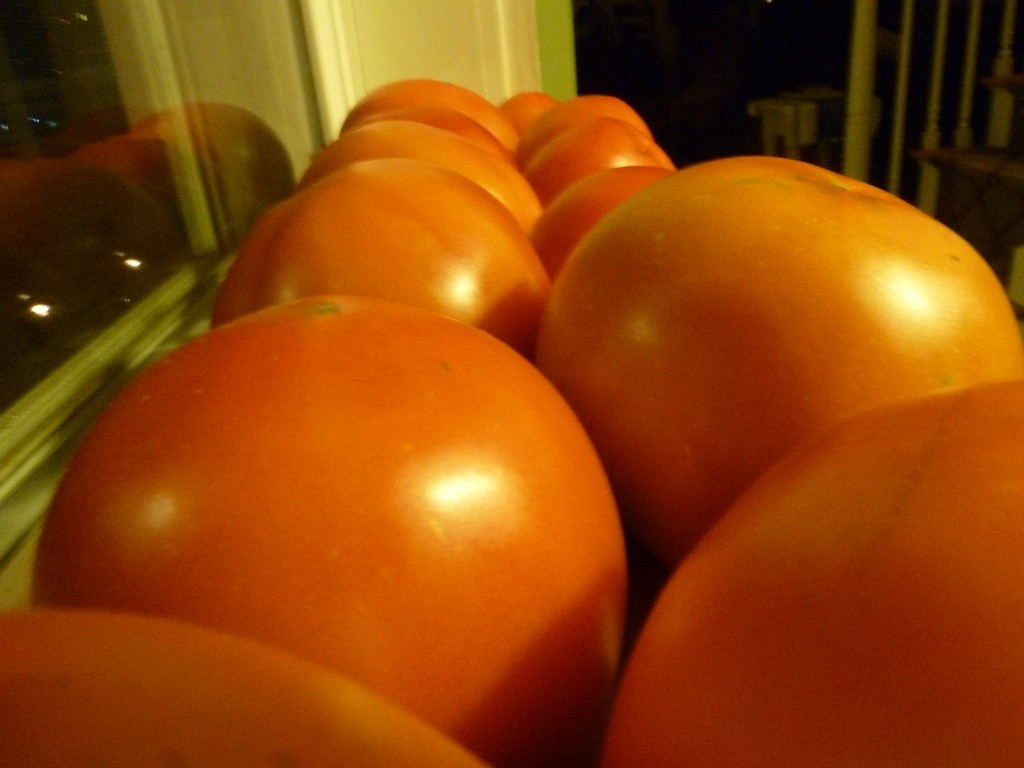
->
[0,77,1024,768]
